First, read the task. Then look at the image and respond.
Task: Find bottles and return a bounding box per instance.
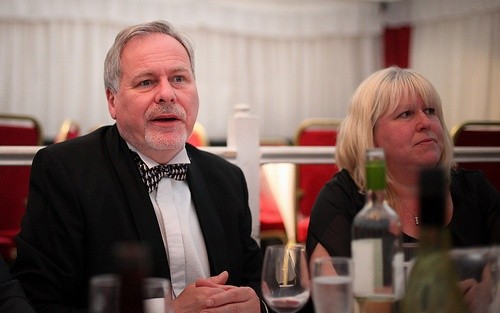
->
[349,147,406,303]
[260,218,285,257]
[405,168,465,313]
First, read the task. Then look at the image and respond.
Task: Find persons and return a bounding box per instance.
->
[16,22,281,313]
[306,68,500,313]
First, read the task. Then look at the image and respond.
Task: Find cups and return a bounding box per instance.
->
[90,275,119,313]
[402,242,418,259]
[129,277,173,313]
[313,255,353,313]
[261,244,311,313]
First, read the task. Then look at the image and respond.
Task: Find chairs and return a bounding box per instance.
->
[294,117,342,246]
[0,114,44,259]
[256,136,295,246]
[449,121,500,198]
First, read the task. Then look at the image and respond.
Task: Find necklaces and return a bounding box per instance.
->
[400,199,419,224]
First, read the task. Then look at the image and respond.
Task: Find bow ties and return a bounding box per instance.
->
[130,149,188,193]
